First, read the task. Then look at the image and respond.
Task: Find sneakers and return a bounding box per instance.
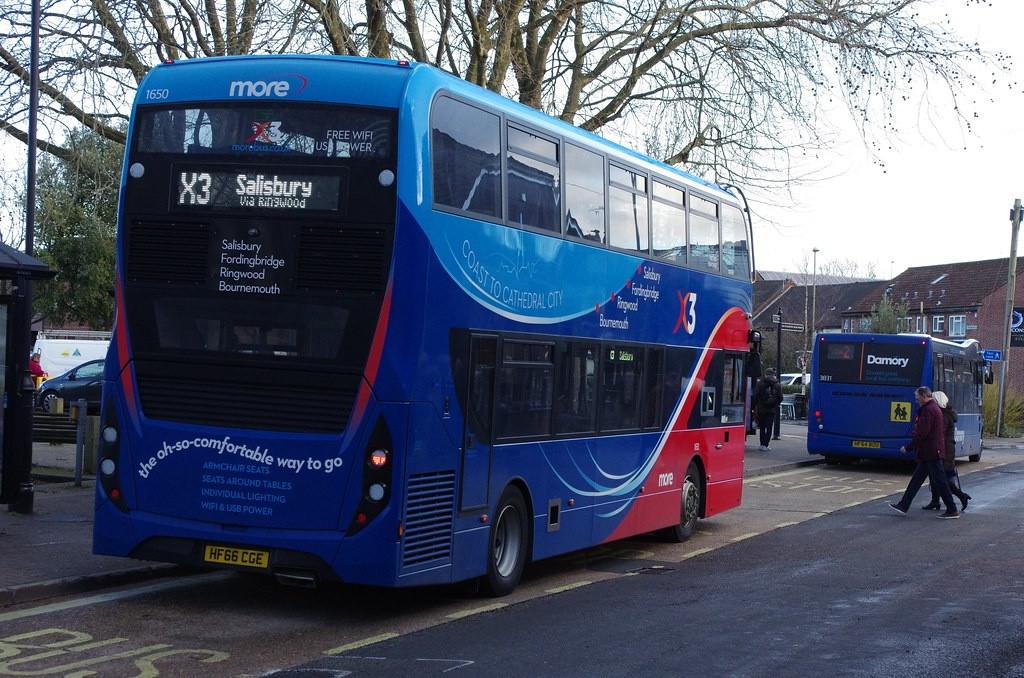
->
[936,510,960,520]
[889,502,909,515]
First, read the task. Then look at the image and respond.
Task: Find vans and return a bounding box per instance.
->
[34,340,111,379]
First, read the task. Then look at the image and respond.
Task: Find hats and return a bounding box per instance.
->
[933,390,949,408]
[765,368,777,376]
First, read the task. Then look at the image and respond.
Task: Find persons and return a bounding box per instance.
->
[887,386,960,520]
[752,368,783,452]
[921,391,971,512]
[29,353,48,386]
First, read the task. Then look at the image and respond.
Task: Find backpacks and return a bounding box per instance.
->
[762,379,780,409]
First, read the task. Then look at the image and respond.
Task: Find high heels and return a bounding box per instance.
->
[922,499,941,510]
[959,494,971,510]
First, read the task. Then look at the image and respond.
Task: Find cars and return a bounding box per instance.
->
[37,360,107,414]
[776,373,811,386]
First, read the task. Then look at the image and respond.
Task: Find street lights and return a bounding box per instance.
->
[812,248,820,353]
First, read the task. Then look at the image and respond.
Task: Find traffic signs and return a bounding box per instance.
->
[772,314,804,333]
[982,350,1002,362]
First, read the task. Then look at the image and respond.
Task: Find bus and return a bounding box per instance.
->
[91,55,763,597]
[808,332,994,471]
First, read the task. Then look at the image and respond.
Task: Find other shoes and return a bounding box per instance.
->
[758,445,762,450]
[763,446,771,450]
